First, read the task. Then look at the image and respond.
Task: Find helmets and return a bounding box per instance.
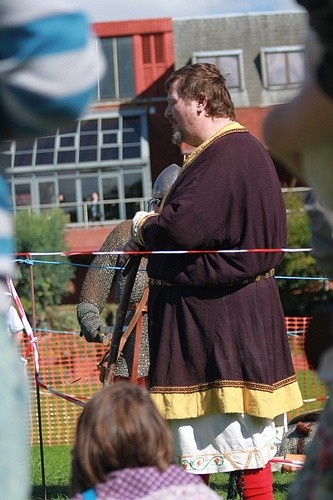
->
[150,164,181,200]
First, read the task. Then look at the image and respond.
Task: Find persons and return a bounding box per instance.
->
[76,164,182,389]
[69,384,222,500]
[130,63,304,500]
[58,194,67,214]
[263,0,333,500]
[88,192,100,220]
[0,0,107,500]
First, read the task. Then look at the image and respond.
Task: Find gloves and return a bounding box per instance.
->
[75,303,107,341]
[131,210,158,247]
